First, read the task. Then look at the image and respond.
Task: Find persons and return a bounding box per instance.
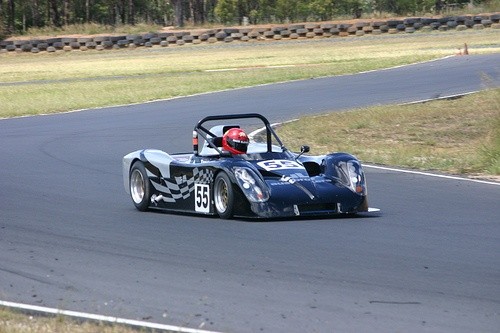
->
[221,128,250,158]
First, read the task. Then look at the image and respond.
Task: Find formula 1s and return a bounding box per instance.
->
[122,113,381,220]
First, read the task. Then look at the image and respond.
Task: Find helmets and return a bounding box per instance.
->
[222,128,249,154]
